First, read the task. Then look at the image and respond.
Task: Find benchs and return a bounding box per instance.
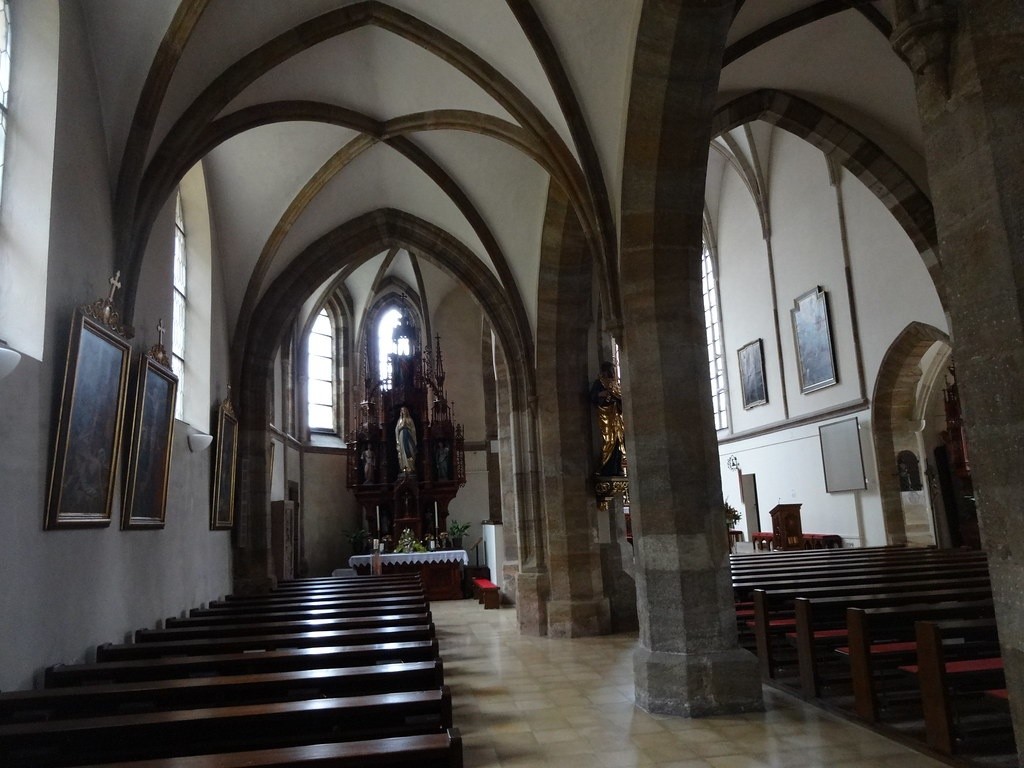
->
[729,545,1009,768]
[473,578,500,609]
[0,570,463,768]
[752,534,842,551]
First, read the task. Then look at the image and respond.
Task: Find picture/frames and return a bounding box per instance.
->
[210,401,240,530]
[737,338,768,410]
[790,285,838,395]
[40,271,132,531]
[113,319,178,531]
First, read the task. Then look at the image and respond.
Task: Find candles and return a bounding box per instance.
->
[435,502,437,527]
[377,506,379,531]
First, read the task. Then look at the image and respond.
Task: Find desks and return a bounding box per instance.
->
[348,550,469,601]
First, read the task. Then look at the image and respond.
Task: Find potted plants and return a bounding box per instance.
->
[724,502,741,523]
[448,520,472,545]
[341,527,369,553]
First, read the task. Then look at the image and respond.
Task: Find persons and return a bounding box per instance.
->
[396,406,418,475]
[360,443,377,484]
[590,362,627,476]
[435,443,452,479]
